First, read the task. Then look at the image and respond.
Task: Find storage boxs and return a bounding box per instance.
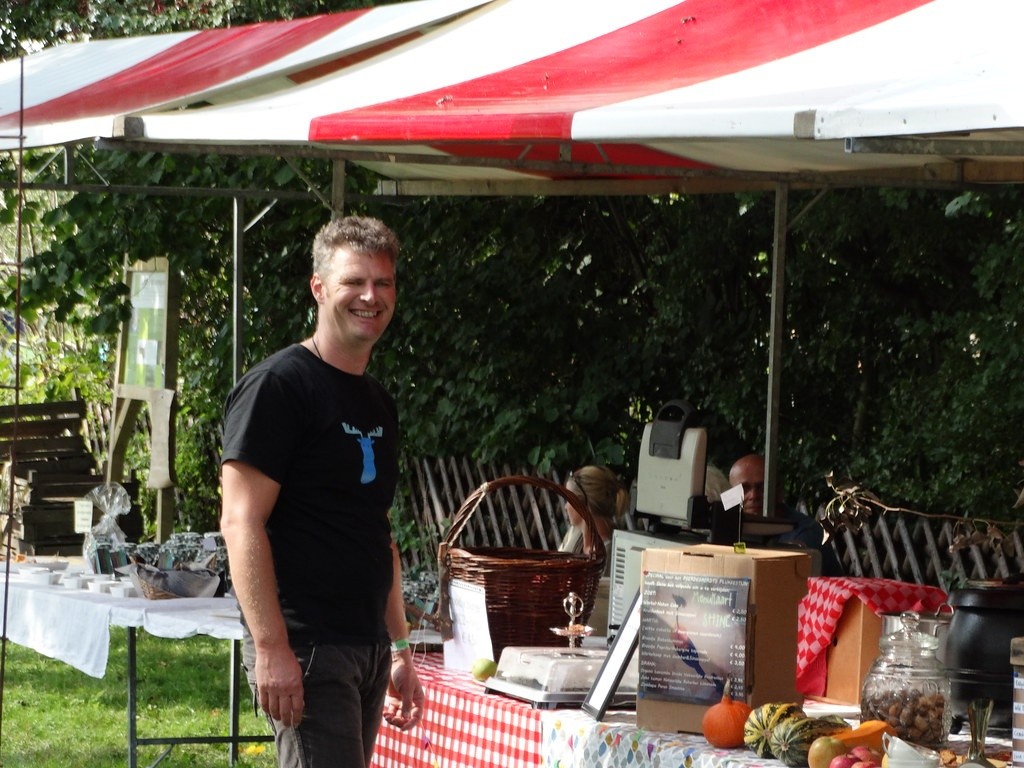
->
[797,577,952,706]
[636,543,812,734]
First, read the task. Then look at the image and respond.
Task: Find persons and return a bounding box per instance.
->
[217,215,425,768]
[730,455,824,548]
[562,465,630,576]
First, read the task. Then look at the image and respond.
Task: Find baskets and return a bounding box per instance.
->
[436,474,607,671]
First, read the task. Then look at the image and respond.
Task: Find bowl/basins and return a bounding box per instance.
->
[0,555,70,571]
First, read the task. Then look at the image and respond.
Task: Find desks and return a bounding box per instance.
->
[0,555,248,768]
[368,650,1024,768]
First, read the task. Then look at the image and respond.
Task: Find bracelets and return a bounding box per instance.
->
[390,640,410,652]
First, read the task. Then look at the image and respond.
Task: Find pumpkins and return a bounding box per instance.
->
[701,679,852,768]
[832,720,898,756]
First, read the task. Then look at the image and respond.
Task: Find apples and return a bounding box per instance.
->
[808,736,882,768]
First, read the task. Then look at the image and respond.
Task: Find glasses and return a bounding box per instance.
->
[568,470,589,511]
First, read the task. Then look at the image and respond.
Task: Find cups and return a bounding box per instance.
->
[0,566,137,598]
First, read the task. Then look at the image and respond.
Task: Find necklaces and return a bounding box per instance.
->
[311,337,322,359]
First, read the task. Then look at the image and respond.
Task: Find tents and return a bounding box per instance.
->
[0,0,1024,533]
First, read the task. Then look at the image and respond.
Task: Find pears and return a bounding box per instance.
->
[472,658,497,681]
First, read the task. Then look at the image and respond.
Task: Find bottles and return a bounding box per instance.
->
[860,587,1024,748]
